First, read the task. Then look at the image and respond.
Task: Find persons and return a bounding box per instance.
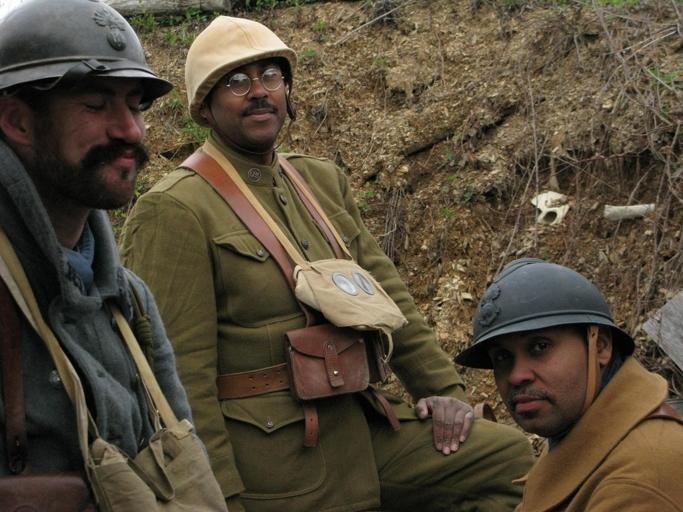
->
[118,14,538,511]
[1,1,200,512]
[452,255,681,512]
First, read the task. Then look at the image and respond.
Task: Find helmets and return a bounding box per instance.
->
[454,258,634,368]
[0,1,172,103]
[183,15,297,127]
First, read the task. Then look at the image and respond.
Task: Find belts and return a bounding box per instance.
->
[216,362,290,401]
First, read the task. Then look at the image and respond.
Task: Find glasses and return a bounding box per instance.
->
[215,69,286,97]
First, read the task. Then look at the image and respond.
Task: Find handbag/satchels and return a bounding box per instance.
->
[283,259,409,407]
[84,420,227,511]
